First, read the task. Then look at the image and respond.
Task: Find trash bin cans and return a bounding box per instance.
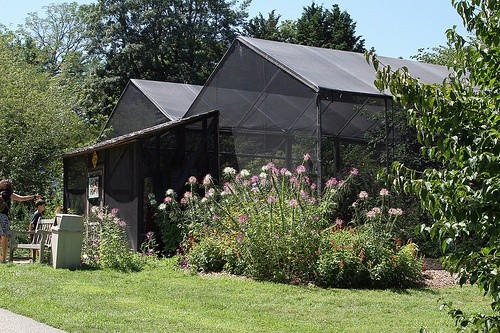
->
[51,214,84,269]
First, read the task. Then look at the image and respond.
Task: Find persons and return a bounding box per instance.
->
[27,199,46,261]
[0,179,41,264]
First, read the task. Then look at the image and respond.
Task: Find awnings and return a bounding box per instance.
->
[61,108,220,160]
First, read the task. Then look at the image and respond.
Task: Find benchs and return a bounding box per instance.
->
[9,216,56,263]
